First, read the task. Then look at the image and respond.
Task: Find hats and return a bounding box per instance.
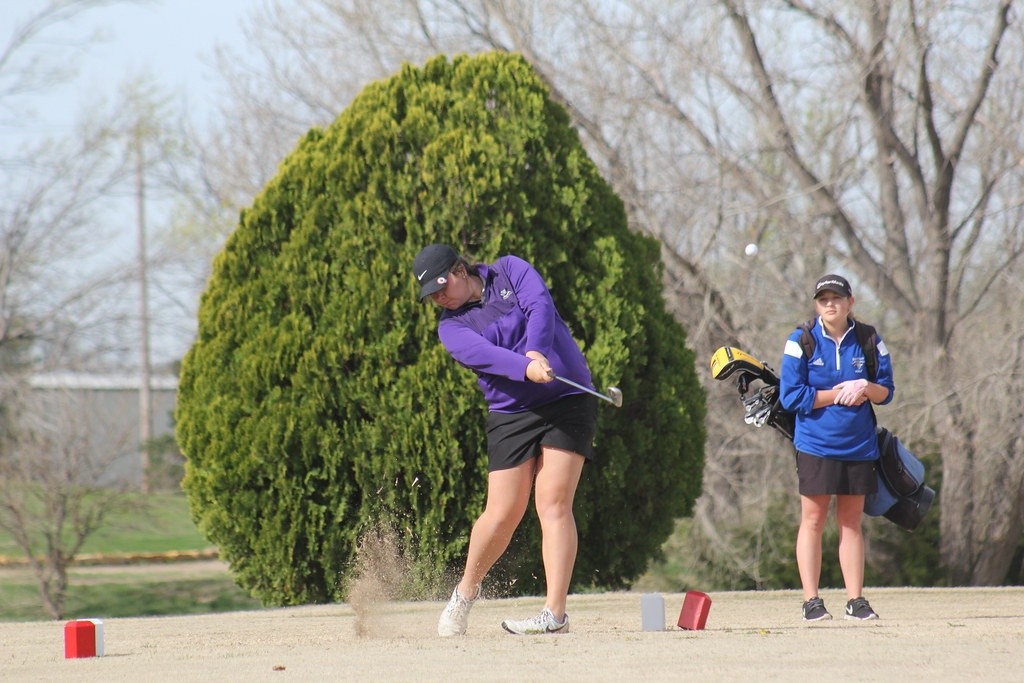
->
[813,275,852,299]
[413,244,458,303]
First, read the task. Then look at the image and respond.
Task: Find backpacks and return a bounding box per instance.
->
[766,386,925,517]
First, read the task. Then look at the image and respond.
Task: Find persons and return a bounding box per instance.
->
[412,242,598,637]
[779,274,894,620]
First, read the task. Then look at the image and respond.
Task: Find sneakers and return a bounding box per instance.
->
[502,607,569,635]
[844,597,879,620]
[438,576,481,637]
[802,597,833,621]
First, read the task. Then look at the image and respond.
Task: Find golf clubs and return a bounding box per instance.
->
[547,371,622,408]
[742,385,779,428]
